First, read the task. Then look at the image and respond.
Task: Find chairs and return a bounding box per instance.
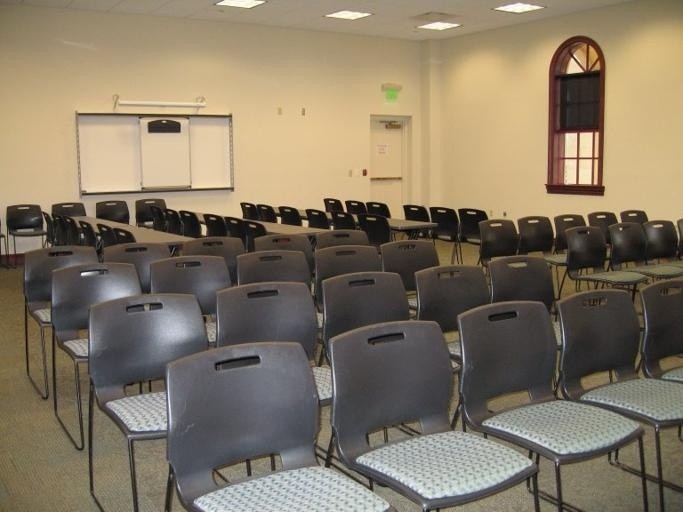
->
[411,263,493,363]
[477,209,682,303]
[555,288,682,511]
[86,293,210,512]
[50,261,143,451]
[20,245,99,399]
[448,298,652,512]
[488,254,566,349]
[0,198,493,343]
[163,341,396,511]
[635,282,682,441]
[318,271,461,436]
[214,279,333,472]
[323,317,542,512]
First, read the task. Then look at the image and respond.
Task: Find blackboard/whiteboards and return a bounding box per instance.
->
[74,110,234,199]
